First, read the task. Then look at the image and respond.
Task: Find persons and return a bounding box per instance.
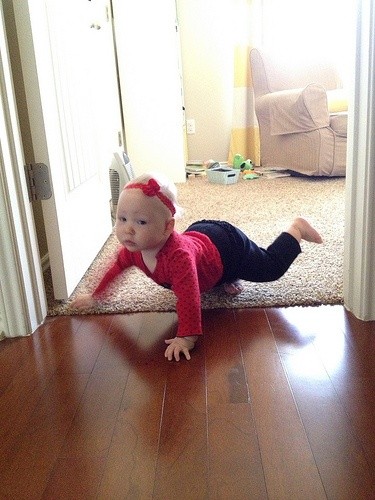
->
[69,173,321,362]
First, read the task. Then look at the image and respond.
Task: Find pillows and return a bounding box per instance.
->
[324,87,348,113]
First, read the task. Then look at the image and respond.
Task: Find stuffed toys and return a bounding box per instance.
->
[233,154,254,170]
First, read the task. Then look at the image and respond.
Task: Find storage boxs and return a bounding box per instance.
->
[207,166,241,184]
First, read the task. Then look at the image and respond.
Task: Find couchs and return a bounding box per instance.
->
[250,34,348,176]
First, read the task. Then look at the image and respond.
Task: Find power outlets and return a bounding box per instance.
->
[187,119,195,135]
